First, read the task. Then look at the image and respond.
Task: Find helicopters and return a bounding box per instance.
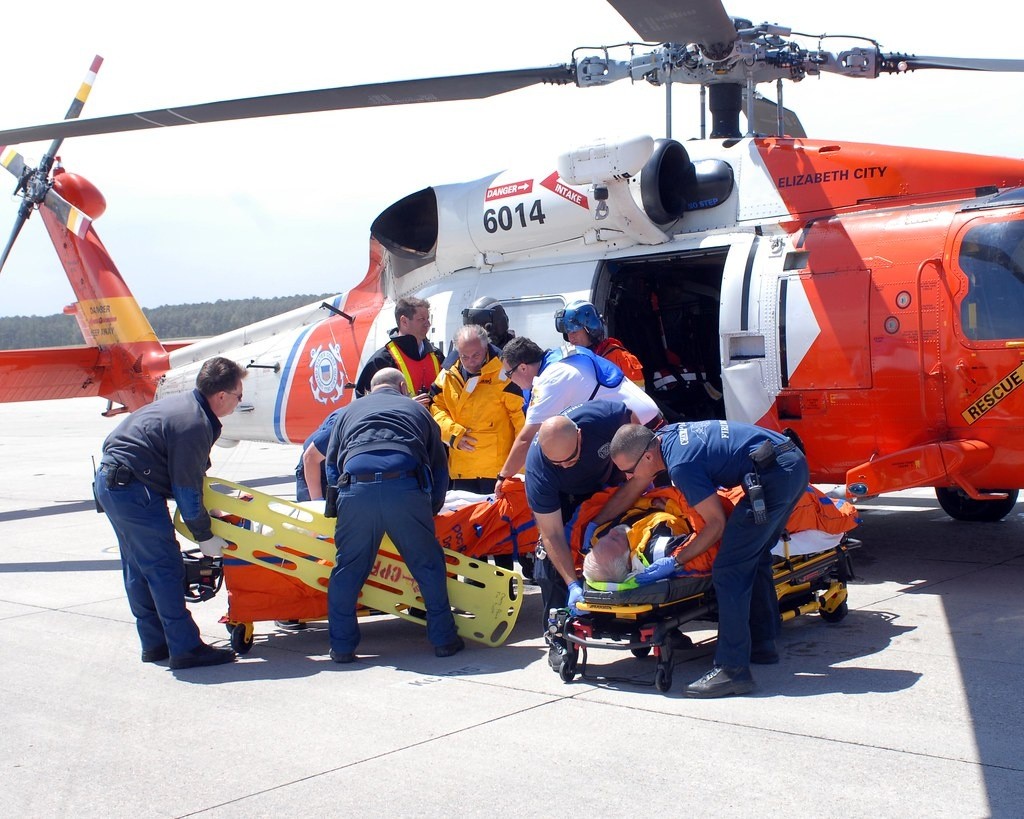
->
[0,0,1024,538]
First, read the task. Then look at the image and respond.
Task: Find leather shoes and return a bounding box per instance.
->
[142,645,168,662]
[169,641,236,669]
[328,648,355,662]
[435,634,465,656]
[548,647,567,671]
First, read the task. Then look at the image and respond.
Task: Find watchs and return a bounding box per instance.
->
[497,473,505,480]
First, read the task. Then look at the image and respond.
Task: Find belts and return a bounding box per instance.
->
[346,469,420,481]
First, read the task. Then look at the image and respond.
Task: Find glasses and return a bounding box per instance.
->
[505,363,527,379]
[222,391,242,400]
[622,449,648,474]
[543,430,578,463]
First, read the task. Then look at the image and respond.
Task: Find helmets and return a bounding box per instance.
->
[555,300,602,342]
[462,296,509,335]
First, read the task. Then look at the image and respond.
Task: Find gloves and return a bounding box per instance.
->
[583,522,599,550]
[635,557,680,585]
[198,535,229,557]
[567,580,591,615]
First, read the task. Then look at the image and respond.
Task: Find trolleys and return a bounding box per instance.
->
[556,519,863,696]
[218,486,541,654]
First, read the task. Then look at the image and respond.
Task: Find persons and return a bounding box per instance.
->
[525,400,642,672]
[576,497,691,583]
[610,419,809,699]
[275,295,668,630]
[94,357,248,669]
[325,367,464,663]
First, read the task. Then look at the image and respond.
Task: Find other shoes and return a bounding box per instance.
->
[275,619,307,630]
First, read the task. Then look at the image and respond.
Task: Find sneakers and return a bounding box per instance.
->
[682,664,754,698]
[750,639,780,664]
